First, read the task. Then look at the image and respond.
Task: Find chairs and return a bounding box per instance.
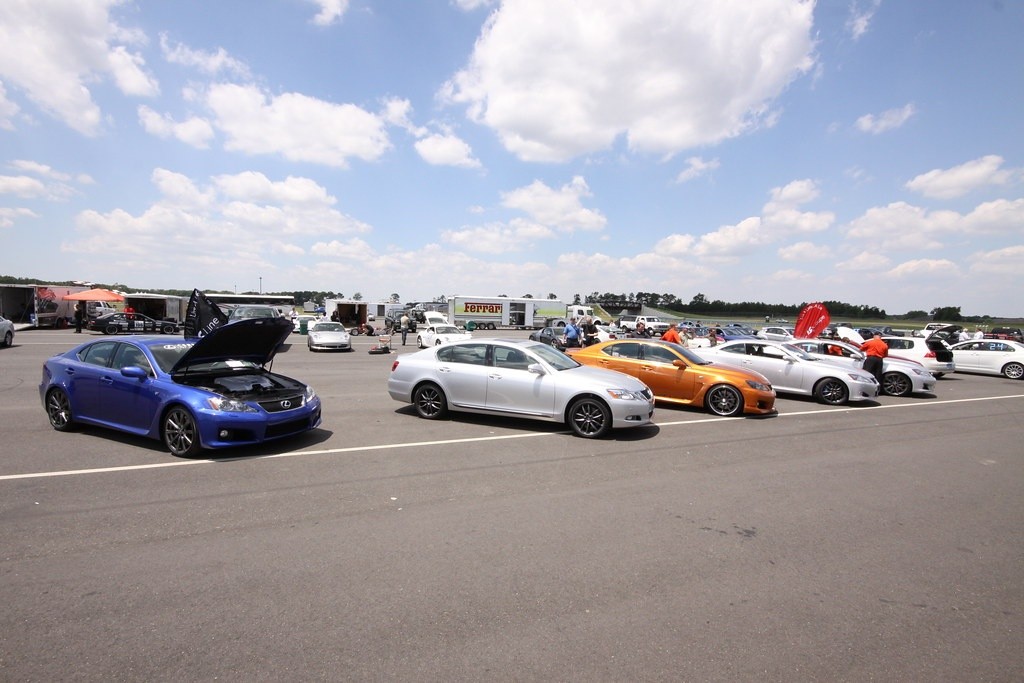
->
[508,352,516,364]
[748,347,766,356]
[379,334,392,350]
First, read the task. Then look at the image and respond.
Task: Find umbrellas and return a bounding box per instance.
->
[61,288,124,322]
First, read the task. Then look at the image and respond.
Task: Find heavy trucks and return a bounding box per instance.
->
[447,296,603,330]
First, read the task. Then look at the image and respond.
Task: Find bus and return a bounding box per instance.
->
[204,294,295,314]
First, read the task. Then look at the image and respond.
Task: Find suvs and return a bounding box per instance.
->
[618,315,670,336]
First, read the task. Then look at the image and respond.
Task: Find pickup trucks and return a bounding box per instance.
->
[92,302,115,314]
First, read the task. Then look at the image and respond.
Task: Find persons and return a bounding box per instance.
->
[563,317,582,347]
[289,307,298,322]
[609,319,616,327]
[959,328,971,342]
[583,317,598,347]
[362,324,375,336]
[860,331,888,397]
[678,326,695,347]
[704,329,717,346]
[716,322,720,327]
[659,323,684,347]
[827,329,850,355]
[400,312,410,345]
[321,312,337,322]
[696,321,702,327]
[973,328,983,349]
[75,305,83,333]
[631,322,651,339]
[124,306,135,319]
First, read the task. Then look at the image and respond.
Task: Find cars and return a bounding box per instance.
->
[984,328,1023,342]
[838,325,963,377]
[563,339,779,419]
[86,314,179,335]
[0,310,15,347]
[758,339,937,397]
[417,310,472,348]
[580,325,627,343]
[217,305,425,352]
[948,338,1023,380]
[530,327,582,348]
[918,322,957,339]
[39,288,322,457]
[386,337,657,438]
[690,339,880,404]
[677,321,914,344]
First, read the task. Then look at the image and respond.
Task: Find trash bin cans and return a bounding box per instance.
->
[467,321,474,331]
[765,316,769,322]
[299,319,308,335]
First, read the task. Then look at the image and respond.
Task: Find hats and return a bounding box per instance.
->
[874,331,882,336]
